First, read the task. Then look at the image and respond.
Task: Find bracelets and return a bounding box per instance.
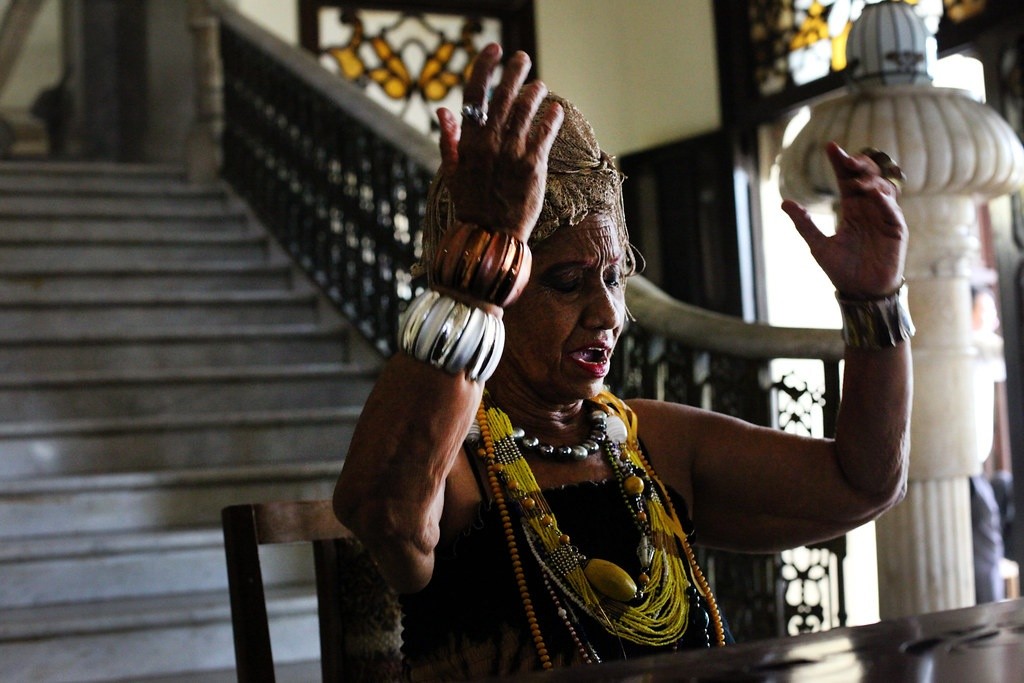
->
[431,221,532,308]
[396,289,505,384]
[834,278,917,351]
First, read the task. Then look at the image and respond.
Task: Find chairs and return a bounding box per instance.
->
[220,499,404,683]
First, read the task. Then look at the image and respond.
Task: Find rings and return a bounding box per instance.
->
[862,145,907,191]
[461,106,483,119]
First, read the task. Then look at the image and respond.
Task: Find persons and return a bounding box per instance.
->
[329,43,914,675]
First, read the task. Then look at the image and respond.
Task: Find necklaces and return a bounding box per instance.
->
[472,387,726,671]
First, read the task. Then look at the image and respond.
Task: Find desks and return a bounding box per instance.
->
[504,598,1024,683]
